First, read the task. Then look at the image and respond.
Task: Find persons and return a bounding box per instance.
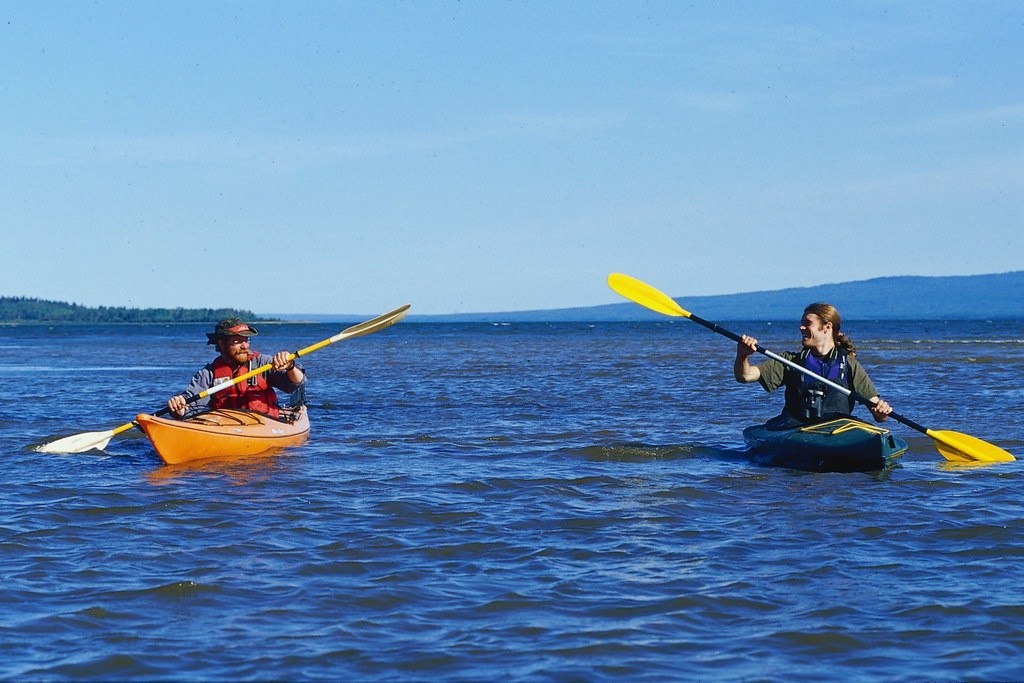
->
[734,303,893,426]
[167,319,308,422]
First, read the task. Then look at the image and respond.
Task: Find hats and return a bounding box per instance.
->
[206,318,258,339]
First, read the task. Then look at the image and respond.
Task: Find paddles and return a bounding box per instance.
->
[32,303,414,452]
[606,272,1017,461]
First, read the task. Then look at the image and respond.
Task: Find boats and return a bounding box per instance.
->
[134,364,313,465]
[744,415,910,471]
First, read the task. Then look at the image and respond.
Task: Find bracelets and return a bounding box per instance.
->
[286,360,296,371]
